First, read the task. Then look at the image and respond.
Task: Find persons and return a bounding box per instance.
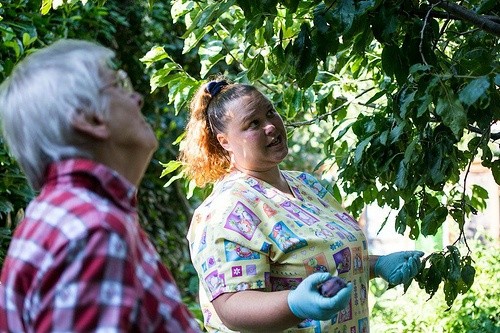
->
[176,75,425,333]
[0,38,203,333]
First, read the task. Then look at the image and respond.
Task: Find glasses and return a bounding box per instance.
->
[97,69,133,94]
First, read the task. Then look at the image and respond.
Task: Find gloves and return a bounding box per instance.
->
[374,250,424,285]
[286,271,352,321]
